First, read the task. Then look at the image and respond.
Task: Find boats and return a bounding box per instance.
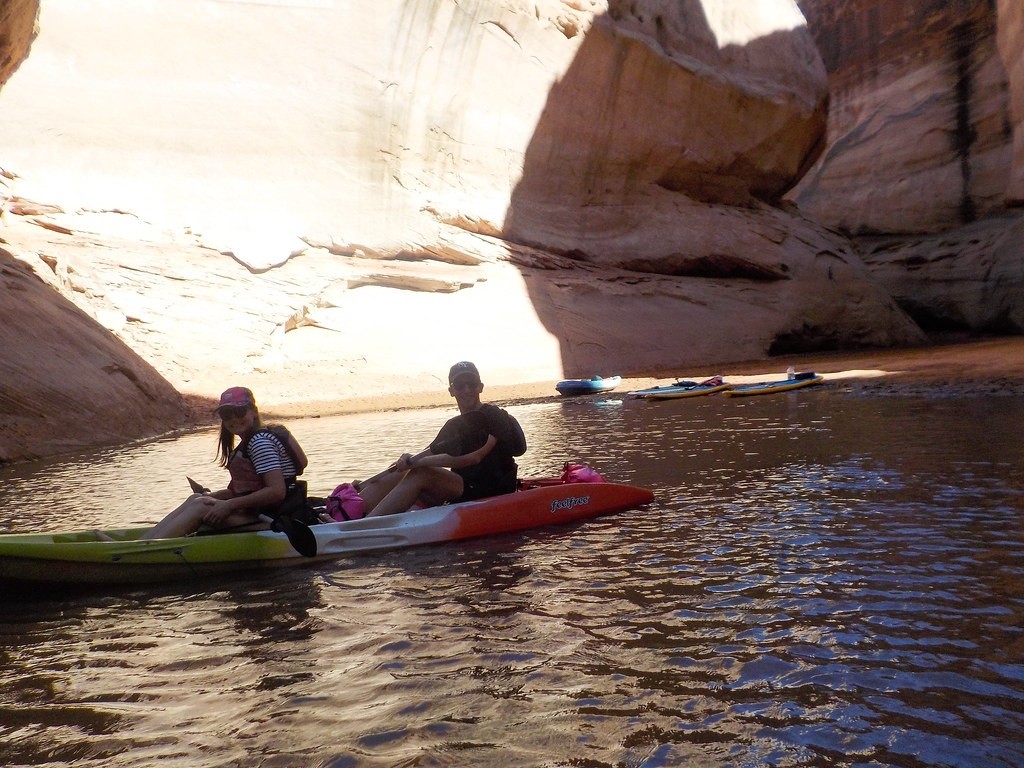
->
[0,473,658,584]
[554,375,622,395]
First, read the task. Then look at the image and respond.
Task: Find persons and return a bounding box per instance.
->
[349,362,518,519]
[93,386,297,542]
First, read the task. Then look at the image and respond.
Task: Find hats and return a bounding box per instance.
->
[449,361,480,386]
[214,387,255,413]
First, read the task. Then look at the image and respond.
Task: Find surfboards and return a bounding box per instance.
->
[645,382,730,399]
[721,374,824,396]
[628,382,697,396]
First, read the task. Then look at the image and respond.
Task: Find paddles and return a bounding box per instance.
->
[354,411,490,491]
[184,474,318,558]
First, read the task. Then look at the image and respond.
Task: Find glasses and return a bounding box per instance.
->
[450,380,481,391]
[218,403,255,421]
